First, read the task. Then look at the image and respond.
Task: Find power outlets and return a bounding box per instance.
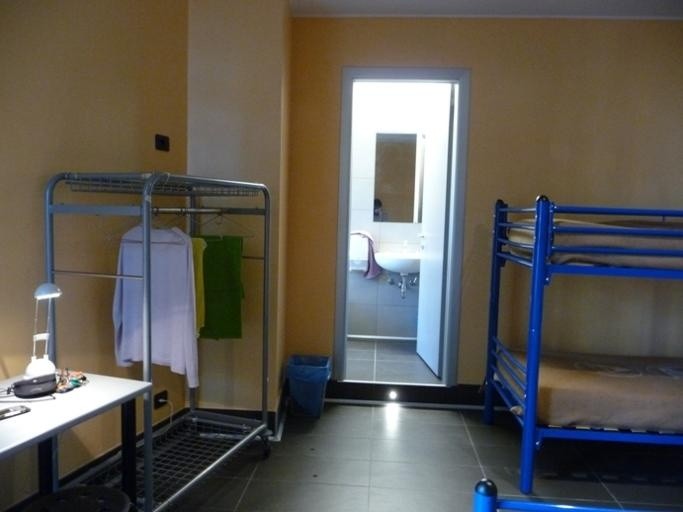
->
[154,390,168,410]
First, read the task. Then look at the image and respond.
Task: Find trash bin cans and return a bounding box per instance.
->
[287,355,332,419]
[23,486,131,512]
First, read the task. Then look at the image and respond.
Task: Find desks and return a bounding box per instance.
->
[0,371,153,512]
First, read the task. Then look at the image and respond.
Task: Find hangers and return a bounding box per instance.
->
[164,207,223,241]
[198,208,255,237]
[118,206,185,245]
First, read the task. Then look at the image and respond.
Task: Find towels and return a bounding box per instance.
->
[350,233,382,279]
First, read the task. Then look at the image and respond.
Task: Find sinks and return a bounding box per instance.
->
[374,251,421,274]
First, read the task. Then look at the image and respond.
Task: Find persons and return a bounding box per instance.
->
[374,199,388,221]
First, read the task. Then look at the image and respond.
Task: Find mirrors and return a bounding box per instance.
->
[372,132,426,224]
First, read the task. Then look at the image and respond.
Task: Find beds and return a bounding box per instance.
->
[481,199,683,495]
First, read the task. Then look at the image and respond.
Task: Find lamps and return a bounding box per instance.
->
[24,282,61,379]
